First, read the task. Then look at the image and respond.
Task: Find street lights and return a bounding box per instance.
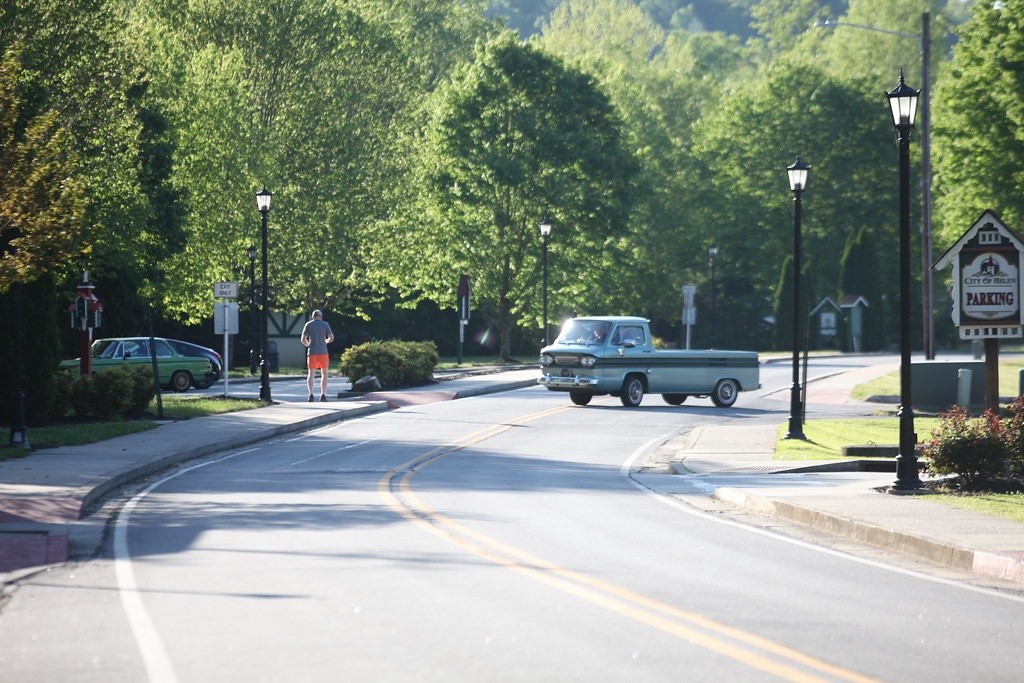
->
[541,214,553,347]
[708,241,718,350]
[815,17,934,361]
[256,184,271,402]
[885,70,925,496]
[787,155,808,438]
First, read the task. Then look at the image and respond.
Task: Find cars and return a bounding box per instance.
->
[88,340,225,389]
[59,338,212,391]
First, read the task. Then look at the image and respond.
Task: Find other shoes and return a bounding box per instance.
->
[308,394,314,402]
[320,395,327,401]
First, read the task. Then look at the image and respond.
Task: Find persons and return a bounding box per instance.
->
[301,310,334,402]
[588,325,607,341]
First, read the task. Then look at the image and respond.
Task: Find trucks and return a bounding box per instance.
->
[538,316,764,408]
